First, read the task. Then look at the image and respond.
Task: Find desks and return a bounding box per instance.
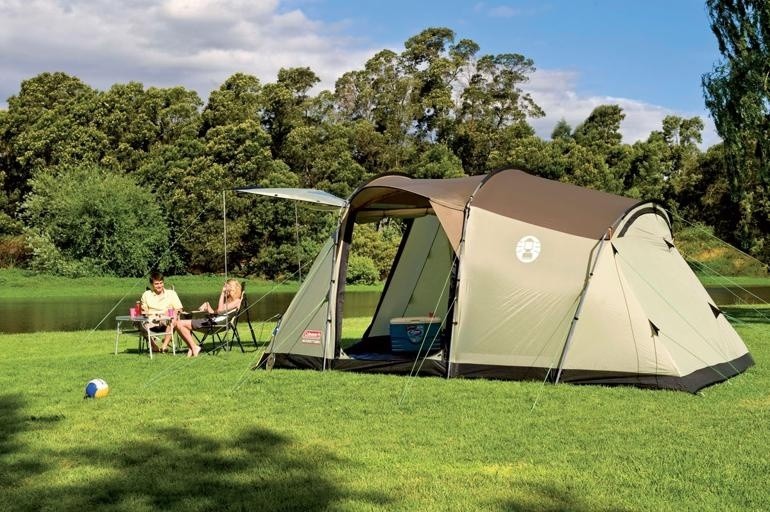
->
[115,315,175,355]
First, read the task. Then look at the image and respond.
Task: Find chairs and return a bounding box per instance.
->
[142,281,258,356]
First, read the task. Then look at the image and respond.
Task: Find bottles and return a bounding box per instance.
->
[135,301,140,317]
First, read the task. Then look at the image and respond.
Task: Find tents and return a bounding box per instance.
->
[237,163,756,395]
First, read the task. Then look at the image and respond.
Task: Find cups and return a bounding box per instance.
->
[168,308,173,317]
[130,308,135,317]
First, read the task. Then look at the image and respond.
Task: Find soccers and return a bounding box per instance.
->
[85,379,110,399]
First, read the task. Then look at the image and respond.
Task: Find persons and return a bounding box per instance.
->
[175,278,242,357]
[139,271,183,354]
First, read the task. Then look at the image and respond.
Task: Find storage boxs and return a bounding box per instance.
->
[390,317,443,355]
